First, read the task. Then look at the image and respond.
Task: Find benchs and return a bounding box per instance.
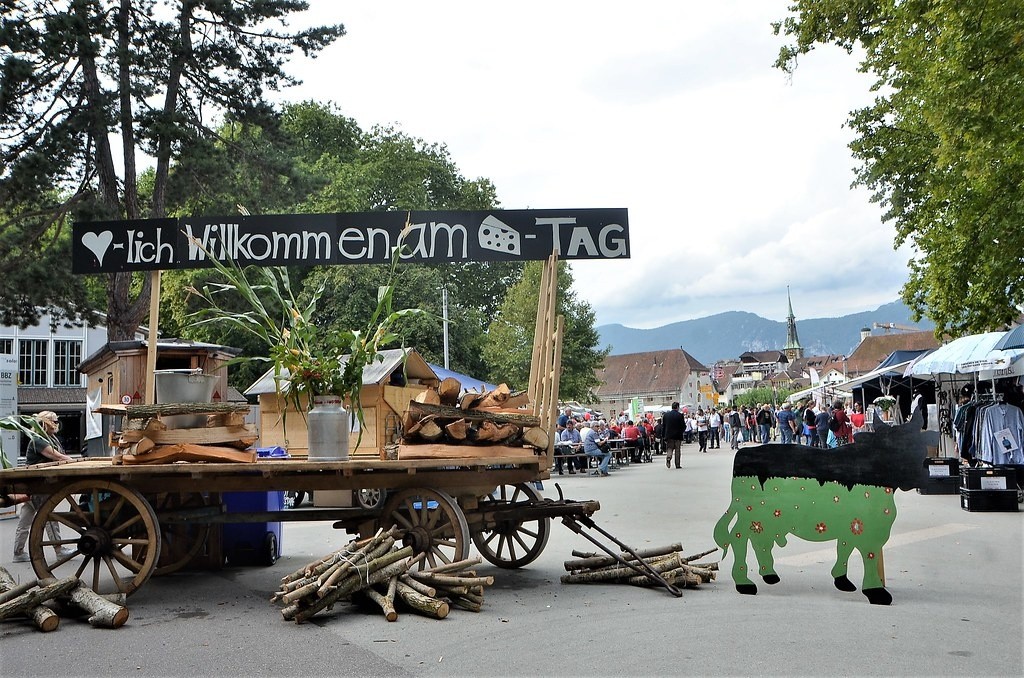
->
[552,448,635,477]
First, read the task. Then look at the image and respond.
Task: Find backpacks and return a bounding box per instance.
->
[828,411,844,431]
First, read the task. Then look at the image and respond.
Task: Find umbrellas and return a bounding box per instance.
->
[902,323,1024,409]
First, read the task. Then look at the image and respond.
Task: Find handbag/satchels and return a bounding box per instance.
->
[596,439,610,453]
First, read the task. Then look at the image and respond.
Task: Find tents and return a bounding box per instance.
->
[426,362,691,425]
[785,346,939,429]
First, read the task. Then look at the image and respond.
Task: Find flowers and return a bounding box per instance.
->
[873,395,897,412]
[182,217,458,454]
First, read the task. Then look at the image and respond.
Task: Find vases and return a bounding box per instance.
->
[306,392,352,462]
[882,411,889,421]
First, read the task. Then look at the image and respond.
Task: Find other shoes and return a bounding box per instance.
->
[704,448,706,452]
[57,547,75,561]
[581,468,586,473]
[12,553,32,563]
[677,466,682,468]
[596,468,611,477]
[709,446,714,449]
[735,447,739,451]
[667,458,670,468]
[699,447,702,452]
[715,446,719,449]
[559,472,564,475]
[569,470,576,473]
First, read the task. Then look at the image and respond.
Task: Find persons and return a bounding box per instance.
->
[539,401,865,476]
[12,411,79,561]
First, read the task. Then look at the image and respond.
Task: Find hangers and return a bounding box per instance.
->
[968,392,1008,405]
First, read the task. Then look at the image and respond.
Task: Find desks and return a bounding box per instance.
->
[865,421,894,432]
[553,438,624,455]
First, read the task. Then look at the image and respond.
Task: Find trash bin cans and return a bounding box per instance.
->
[220,446,288,567]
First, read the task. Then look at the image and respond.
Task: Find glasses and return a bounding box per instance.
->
[54,421,60,424]
[595,426,599,428]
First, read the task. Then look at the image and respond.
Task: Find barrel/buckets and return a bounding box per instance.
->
[152,368,222,431]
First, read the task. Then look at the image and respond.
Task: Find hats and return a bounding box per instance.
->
[783,403,790,409]
